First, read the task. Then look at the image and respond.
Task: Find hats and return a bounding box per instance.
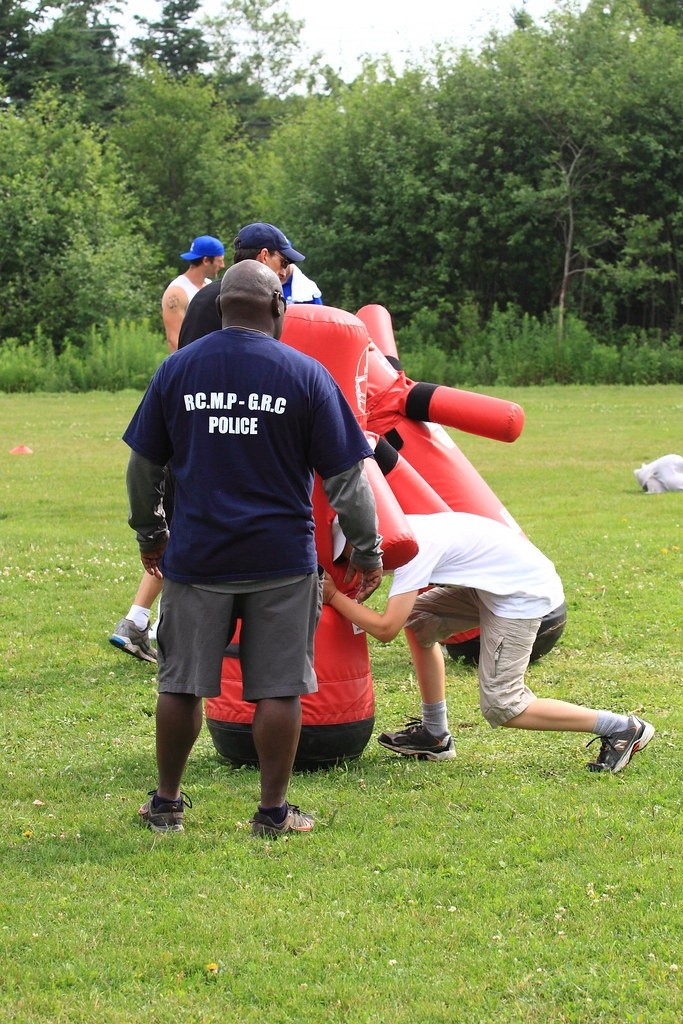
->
[234,223,304,261]
[332,512,346,563]
[180,236,224,260]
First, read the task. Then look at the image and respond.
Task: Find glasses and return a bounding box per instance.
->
[262,248,289,269]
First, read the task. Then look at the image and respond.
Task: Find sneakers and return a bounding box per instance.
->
[148,618,159,640]
[379,716,456,761]
[110,618,158,664]
[138,789,184,834]
[586,714,655,774]
[251,801,316,841]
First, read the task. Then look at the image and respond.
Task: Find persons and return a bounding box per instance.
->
[321,511,655,776]
[110,222,306,667]
[119,256,384,844]
[163,236,225,349]
[276,260,321,304]
